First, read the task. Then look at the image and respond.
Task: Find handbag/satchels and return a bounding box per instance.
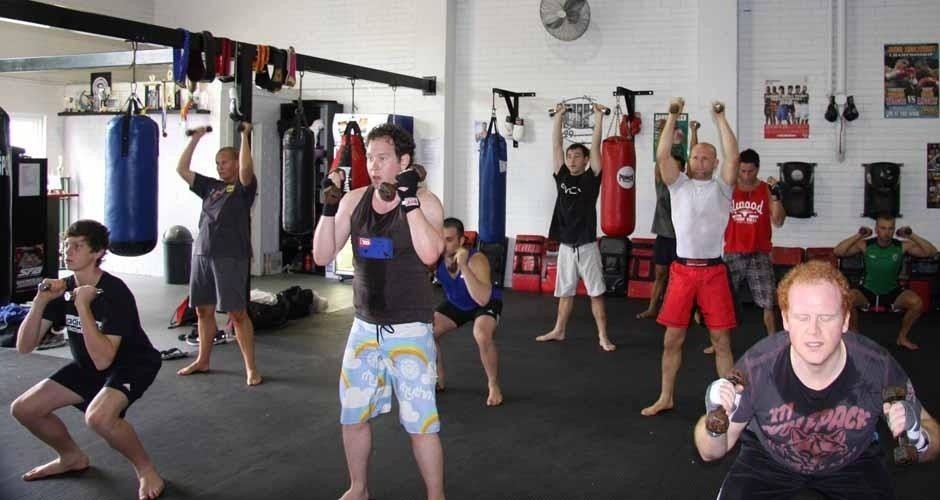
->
[245,283,313,337]
[170,295,199,329]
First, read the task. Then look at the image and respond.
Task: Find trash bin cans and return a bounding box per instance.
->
[162,225,193,284]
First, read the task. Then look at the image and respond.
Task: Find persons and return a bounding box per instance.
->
[433,218,504,407]
[175,119,261,386]
[703,149,785,355]
[535,102,617,351]
[636,119,702,324]
[693,260,940,500]
[312,126,444,500]
[833,211,937,351]
[764,85,809,126]
[884,59,938,97]
[10,219,165,500]
[640,98,741,417]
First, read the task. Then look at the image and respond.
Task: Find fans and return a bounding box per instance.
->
[539,0,591,42]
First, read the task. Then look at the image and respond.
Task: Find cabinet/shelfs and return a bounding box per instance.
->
[277,99,344,276]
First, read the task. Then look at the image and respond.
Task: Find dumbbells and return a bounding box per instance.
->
[38,277,68,291]
[234,120,254,132]
[704,370,744,434]
[588,103,610,116]
[549,104,572,116]
[320,167,348,204]
[670,102,686,114]
[186,127,212,135]
[65,289,104,302]
[881,384,919,467]
[447,245,472,272]
[714,101,724,113]
[378,166,426,201]
[896,226,912,236]
[859,227,873,236]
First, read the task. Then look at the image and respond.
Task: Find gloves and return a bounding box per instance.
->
[881,398,931,457]
[703,376,744,438]
[395,167,421,215]
[319,167,346,217]
